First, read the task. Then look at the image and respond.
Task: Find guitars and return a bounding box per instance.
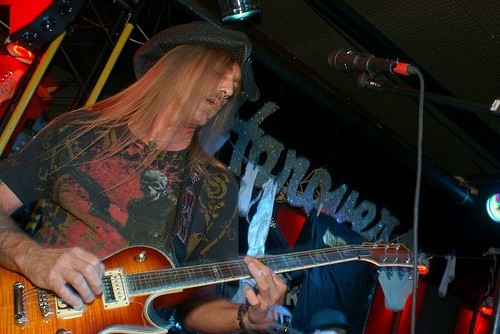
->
[0,241,429,334]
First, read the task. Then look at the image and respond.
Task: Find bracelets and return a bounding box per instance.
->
[238,304,251,334]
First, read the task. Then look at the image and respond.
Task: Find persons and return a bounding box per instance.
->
[0,19,289,334]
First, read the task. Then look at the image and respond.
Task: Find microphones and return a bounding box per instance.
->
[326,48,418,76]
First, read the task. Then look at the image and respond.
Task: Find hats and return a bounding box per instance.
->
[133,21,251,80]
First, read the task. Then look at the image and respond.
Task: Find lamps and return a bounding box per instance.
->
[220,0,258,22]
